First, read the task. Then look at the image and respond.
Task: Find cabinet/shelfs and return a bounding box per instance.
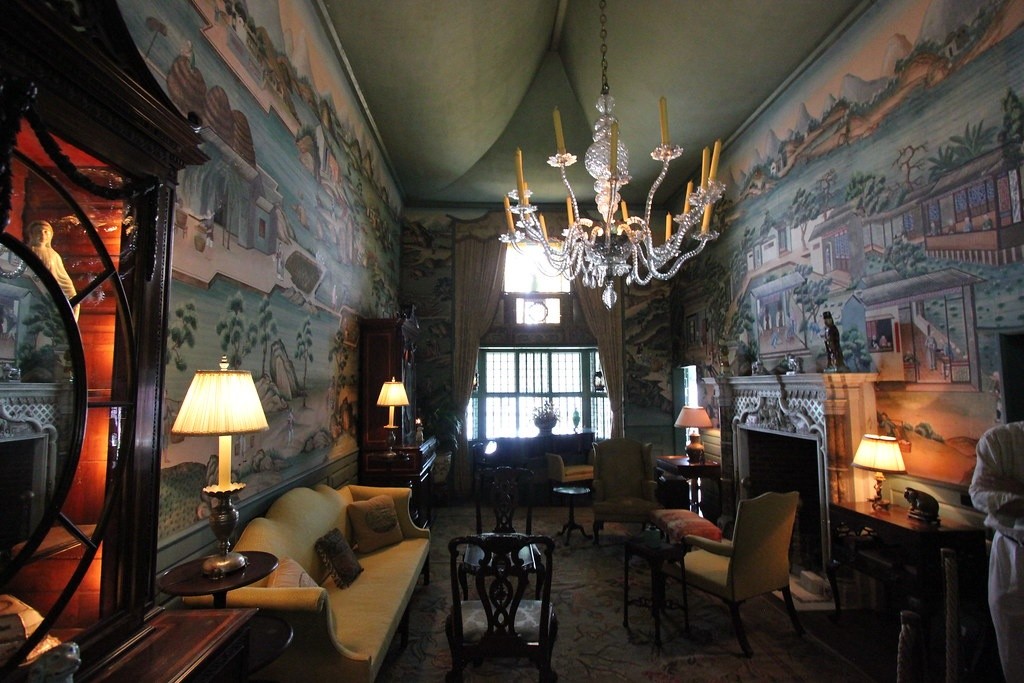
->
[354,304,439,529]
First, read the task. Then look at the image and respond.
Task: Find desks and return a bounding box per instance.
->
[828,498,993,660]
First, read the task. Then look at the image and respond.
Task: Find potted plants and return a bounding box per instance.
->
[416,356,466,481]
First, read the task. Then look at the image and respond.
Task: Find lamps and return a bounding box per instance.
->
[170,355,270,576]
[850,434,907,509]
[502,0,723,310]
[376,377,410,428]
[673,406,713,463]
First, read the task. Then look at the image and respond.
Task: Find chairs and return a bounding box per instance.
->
[443,532,560,682]
[592,438,656,547]
[677,490,804,657]
[457,466,546,602]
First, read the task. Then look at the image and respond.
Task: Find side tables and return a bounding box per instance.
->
[155,553,294,680]
[653,455,723,520]
[553,487,594,547]
[355,462,429,530]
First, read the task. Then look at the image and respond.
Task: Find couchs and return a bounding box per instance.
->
[181,481,432,683]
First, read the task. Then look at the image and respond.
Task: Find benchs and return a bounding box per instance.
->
[472,431,598,502]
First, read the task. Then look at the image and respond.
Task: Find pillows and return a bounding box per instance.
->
[313,527,364,589]
[268,554,337,636]
[347,495,404,554]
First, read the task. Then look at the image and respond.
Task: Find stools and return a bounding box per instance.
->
[623,537,690,650]
[653,509,722,603]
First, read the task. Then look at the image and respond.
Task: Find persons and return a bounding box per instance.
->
[25,220,82,323]
[967,421,1023,683]
